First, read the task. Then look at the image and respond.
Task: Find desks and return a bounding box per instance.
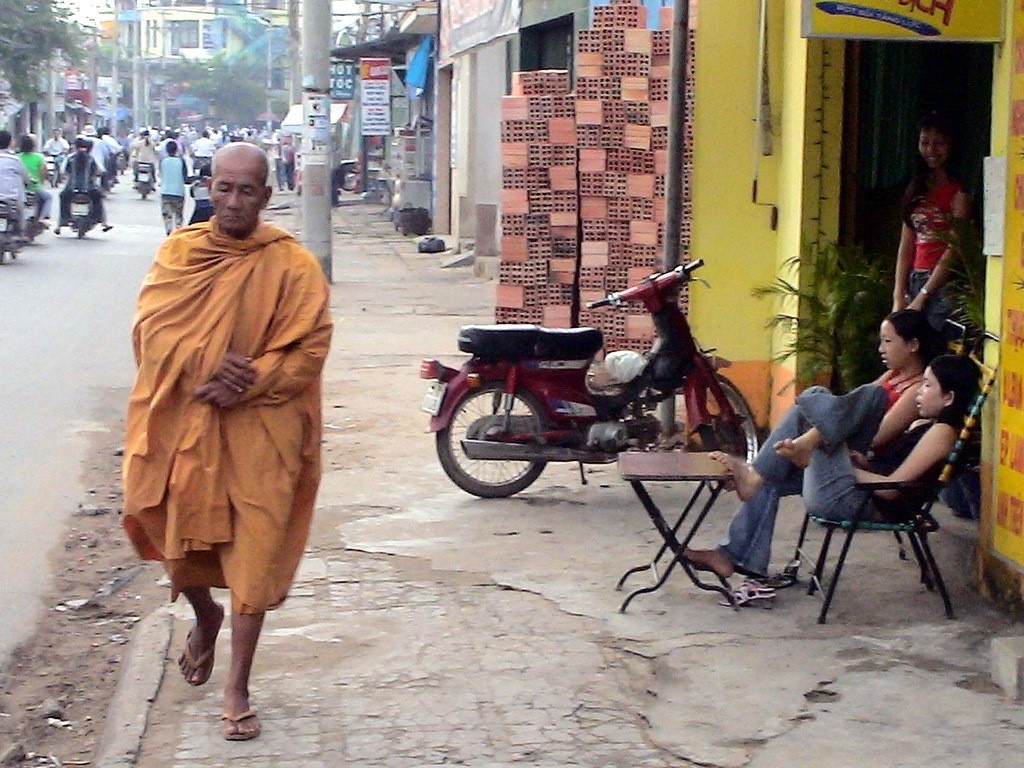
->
[611,447,739,613]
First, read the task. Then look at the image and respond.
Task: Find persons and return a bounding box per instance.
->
[14,134,52,231]
[53,139,111,235]
[0,129,31,243]
[156,142,188,237]
[890,112,977,520]
[682,308,941,580]
[709,353,978,523]
[117,142,333,743]
[42,124,297,193]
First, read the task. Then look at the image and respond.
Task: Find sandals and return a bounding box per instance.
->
[718,558,800,609]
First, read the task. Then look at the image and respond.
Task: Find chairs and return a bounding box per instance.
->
[790,318,1002,625]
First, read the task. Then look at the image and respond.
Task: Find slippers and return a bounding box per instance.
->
[178,603,224,682]
[220,702,260,741]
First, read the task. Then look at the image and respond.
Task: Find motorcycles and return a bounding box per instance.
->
[118,148,128,175]
[94,159,110,197]
[1,179,36,266]
[42,151,68,188]
[133,155,156,200]
[421,258,759,498]
[68,172,106,238]
[24,169,55,241]
[192,155,212,174]
[107,151,123,187]
